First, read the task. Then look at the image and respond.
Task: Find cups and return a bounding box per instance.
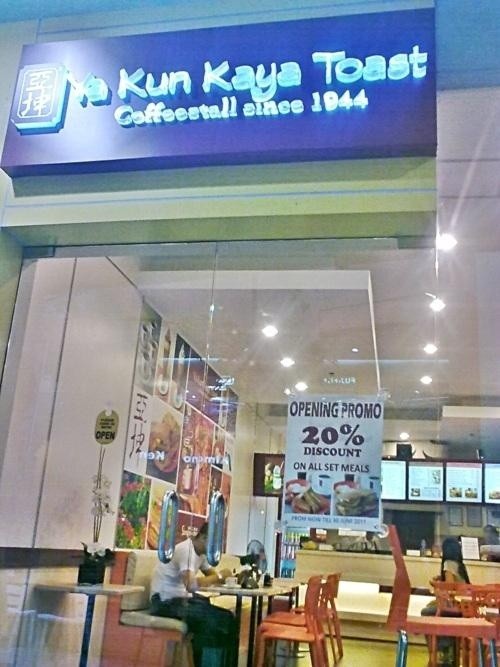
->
[225,577,237,586]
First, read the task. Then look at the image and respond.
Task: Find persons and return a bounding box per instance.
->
[422,539,470,665]
[149,523,238,667]
[482,525,500,561]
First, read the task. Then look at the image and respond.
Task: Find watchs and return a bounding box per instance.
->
[216,573,224,582]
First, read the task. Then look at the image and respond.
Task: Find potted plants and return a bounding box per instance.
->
[69,542,113,584]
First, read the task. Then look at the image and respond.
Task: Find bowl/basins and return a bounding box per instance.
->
[286,479,312,495]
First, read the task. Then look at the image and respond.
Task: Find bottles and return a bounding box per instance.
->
[273,465,281,490]
[264,572,271,586]
[282,531,310,578]
[421,539,427,556]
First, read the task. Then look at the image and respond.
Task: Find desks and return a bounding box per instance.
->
[37,584,146,666]
[196,578,302,667]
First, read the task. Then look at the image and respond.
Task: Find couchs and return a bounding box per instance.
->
[100,547,268,667]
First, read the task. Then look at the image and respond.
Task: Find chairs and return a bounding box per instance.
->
[256,574,343,667]
[388,523,500,666]
